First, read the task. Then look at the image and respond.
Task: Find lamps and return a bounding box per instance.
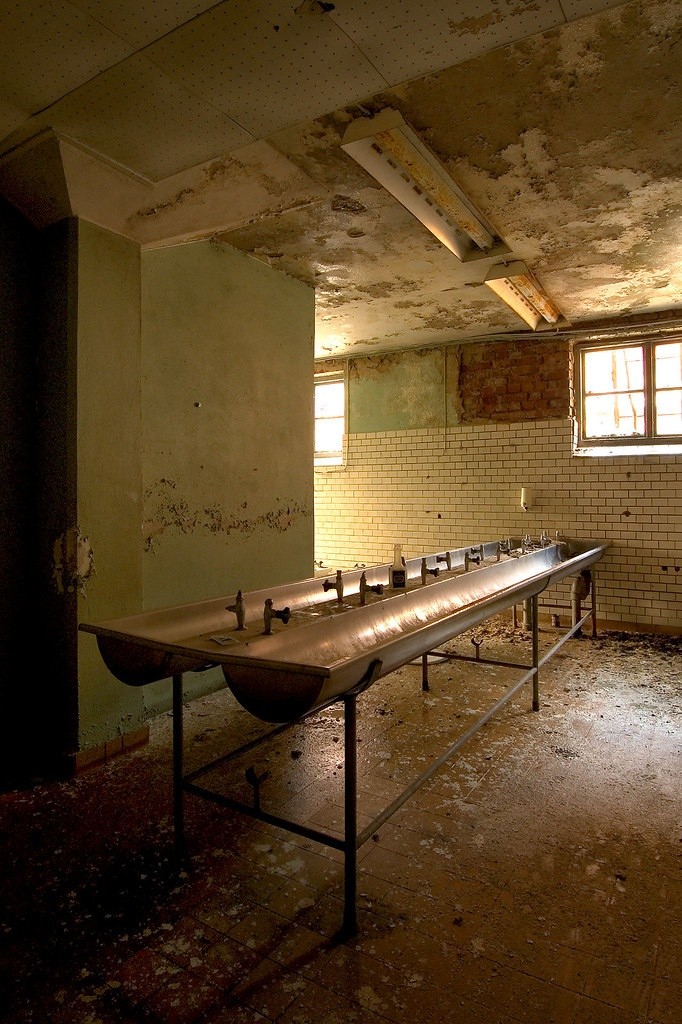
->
[520,487,532,513]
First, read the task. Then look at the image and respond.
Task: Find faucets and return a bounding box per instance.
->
[497,531,552,561]
[465,552,480,571]
[360,571,384,606]
[225,590,249,630]
[471,544,484,561]
[261,598,292,635]
[421,558,439,585]
[322,570,345,605]
[436,552,451,571]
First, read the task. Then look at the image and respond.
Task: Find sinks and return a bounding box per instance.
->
[220,539,614,724]
[77,537,556,687]
